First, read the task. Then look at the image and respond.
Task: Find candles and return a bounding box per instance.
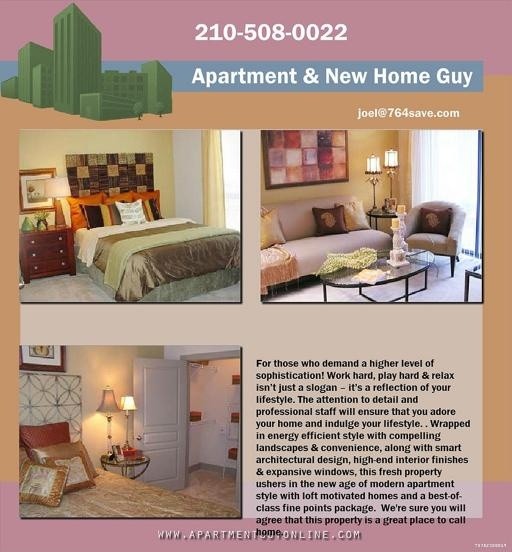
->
[397,203,406,216]
[390,217,401,229]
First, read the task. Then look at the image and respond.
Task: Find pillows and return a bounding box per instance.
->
[261,205,287,250]
[417,206,455,236]
[334,200,368,234]
[19,420,100,508]
[65,189,164,234]
[311,202,348,239]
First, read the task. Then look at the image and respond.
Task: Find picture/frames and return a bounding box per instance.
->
[19,344,70,372]
[18,167,59,216]
[261,130,351,191]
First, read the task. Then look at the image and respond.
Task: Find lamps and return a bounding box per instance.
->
[94,385,124,458]
[362,151,384,215]
[45,177,74,229]
[382,147,400,211]
[119,393,140,454]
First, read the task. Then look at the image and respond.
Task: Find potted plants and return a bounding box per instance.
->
[34,209,52,230]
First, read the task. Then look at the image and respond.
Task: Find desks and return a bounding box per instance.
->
[100,449,153,481]
[367,207,406,231]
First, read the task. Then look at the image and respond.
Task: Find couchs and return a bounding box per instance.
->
[260,197,397,297]
[397,198,469,282]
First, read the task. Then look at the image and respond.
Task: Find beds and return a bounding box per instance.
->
[67,188,240,303]
[19,373,243,517]
[318,253,433,303]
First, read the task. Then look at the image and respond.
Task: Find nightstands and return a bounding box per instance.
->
[20,225,77,285]
